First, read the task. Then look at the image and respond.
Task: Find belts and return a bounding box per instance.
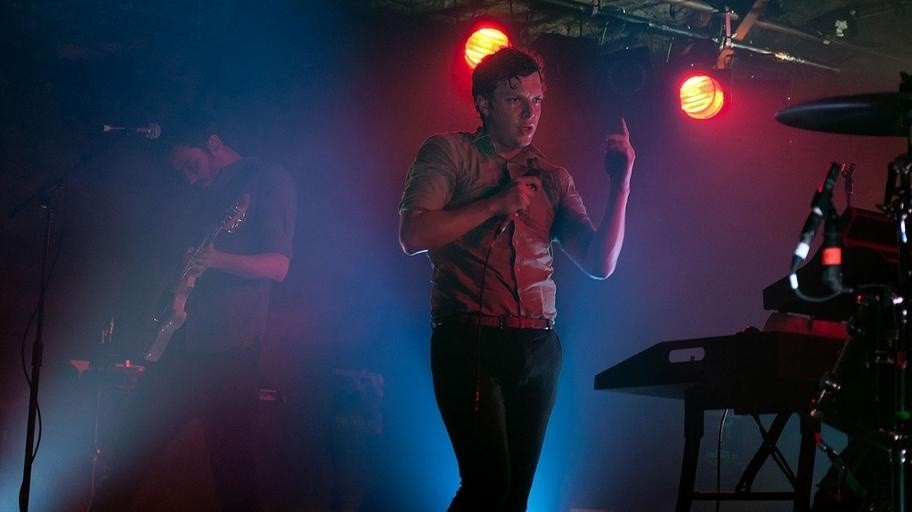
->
[451,312,555,331]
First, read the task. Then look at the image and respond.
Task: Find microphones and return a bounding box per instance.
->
[791,162,840,275]
[493,168,543,241]
[82,121,160,140]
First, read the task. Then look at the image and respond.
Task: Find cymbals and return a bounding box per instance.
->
[774,91,910,136]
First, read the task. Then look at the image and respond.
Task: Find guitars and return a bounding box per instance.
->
[136,191,252,364]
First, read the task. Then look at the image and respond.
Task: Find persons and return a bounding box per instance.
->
[161,101,294,510]
[397,46,638,510]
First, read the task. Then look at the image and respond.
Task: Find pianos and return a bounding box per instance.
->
[595,329,844,408]
[764,204,911,317]
